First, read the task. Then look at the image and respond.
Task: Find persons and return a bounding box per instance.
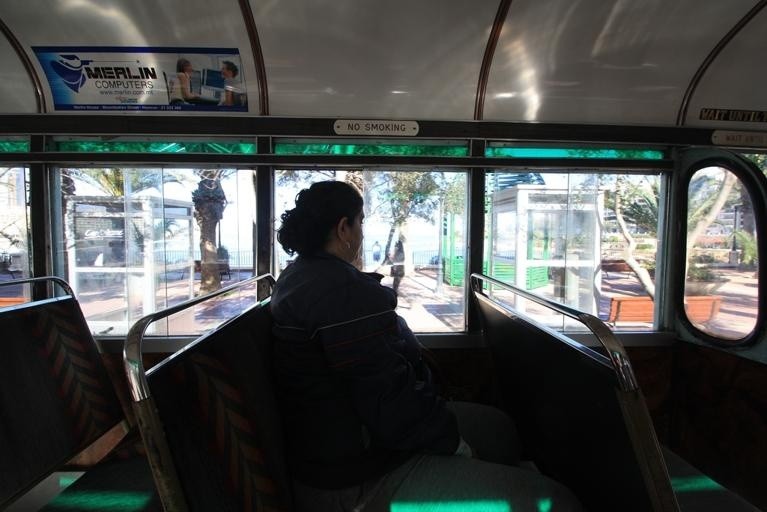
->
[6,239,27,279]
[371,240,382,269]
[386,240,407,298]
[267,180,430,511]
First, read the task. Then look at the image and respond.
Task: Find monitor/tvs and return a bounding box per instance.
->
[203,69,225,91]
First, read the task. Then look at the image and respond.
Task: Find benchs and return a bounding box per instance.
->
[465,271,767,512]
[603,296,724,335]
[1,274,162,512]
[120,274,297,512]
